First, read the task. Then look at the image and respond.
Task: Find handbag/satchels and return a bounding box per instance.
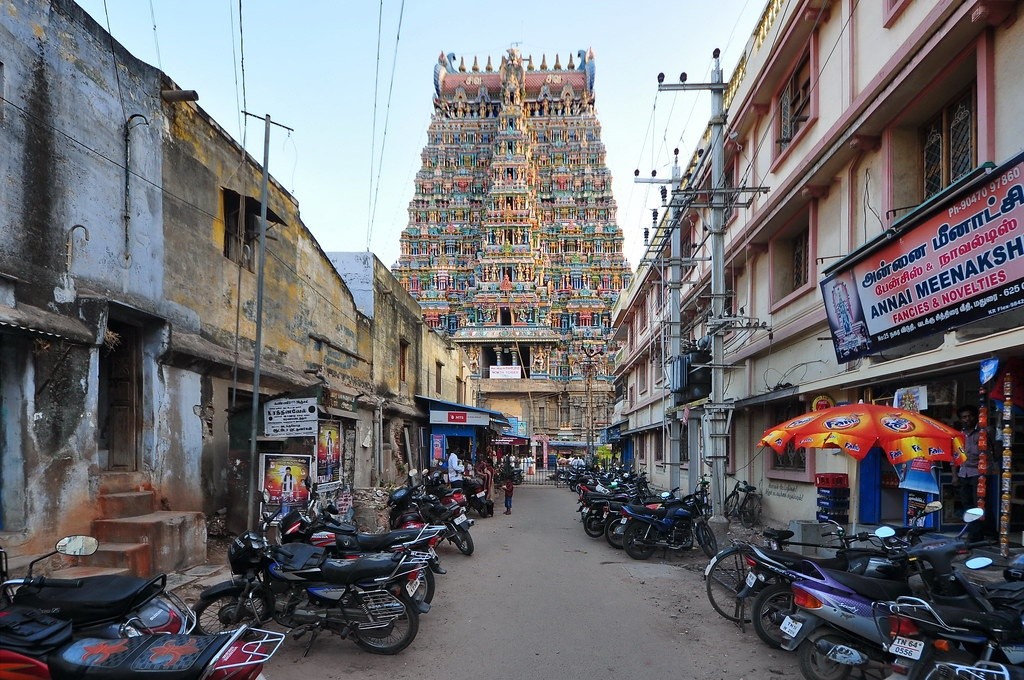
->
[0,607,73,657]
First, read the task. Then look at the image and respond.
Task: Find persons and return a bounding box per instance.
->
[448,444,465,489]
[504,453,535,474]
[393,67,633,376]
[502,477,514,515]
[485,458,494,498]
[558,455,585,466]
[956,407,990,543]
[326,431,334,480]
[281,467,297,513]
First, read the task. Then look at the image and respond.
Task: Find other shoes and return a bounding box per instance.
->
[503,510,511,515]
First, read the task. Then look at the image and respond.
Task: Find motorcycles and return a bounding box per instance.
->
[548,458,1024,679]
[0,463,493,680]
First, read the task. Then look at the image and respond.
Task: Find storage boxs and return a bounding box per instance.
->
[815,473,851,524]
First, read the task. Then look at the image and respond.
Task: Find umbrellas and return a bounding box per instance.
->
[756,402,966,551]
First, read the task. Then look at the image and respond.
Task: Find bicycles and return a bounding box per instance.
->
[703,514,852,635]
[720,471,764,527]
